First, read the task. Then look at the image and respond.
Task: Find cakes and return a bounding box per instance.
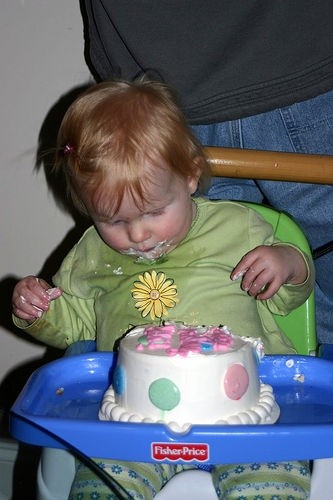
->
[98,321,281,434]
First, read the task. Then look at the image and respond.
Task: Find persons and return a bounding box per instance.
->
[78,0,333,362]
[10,78,316,499]
